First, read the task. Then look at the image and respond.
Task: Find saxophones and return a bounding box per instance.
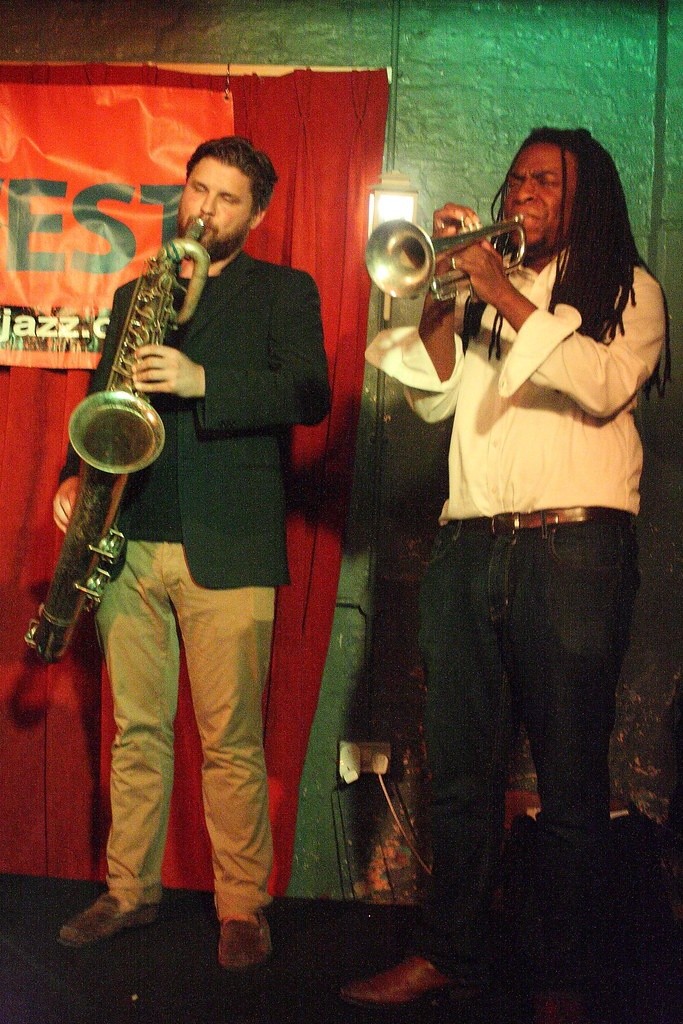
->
[23,213,214,664]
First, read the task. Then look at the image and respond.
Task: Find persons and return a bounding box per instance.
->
[340,127,671,1023]
[46,134,334,973]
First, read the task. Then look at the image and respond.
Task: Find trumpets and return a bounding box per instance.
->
[364,211,523,302]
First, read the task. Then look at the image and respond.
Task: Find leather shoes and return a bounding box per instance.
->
[529,985,592,1024]
[218,908,273,972]
[55,893,158,948]
[337,953,463,1010]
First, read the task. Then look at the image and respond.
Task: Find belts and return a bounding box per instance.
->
[448,507,636,535]
